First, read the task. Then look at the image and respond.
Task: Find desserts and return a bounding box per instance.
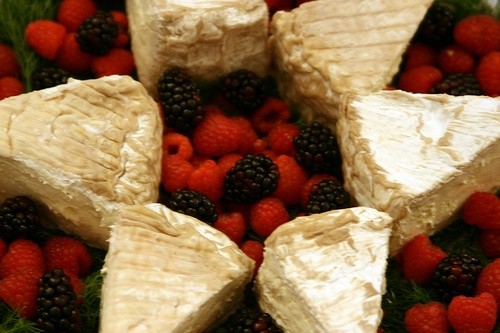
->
[0,0,500,333]
